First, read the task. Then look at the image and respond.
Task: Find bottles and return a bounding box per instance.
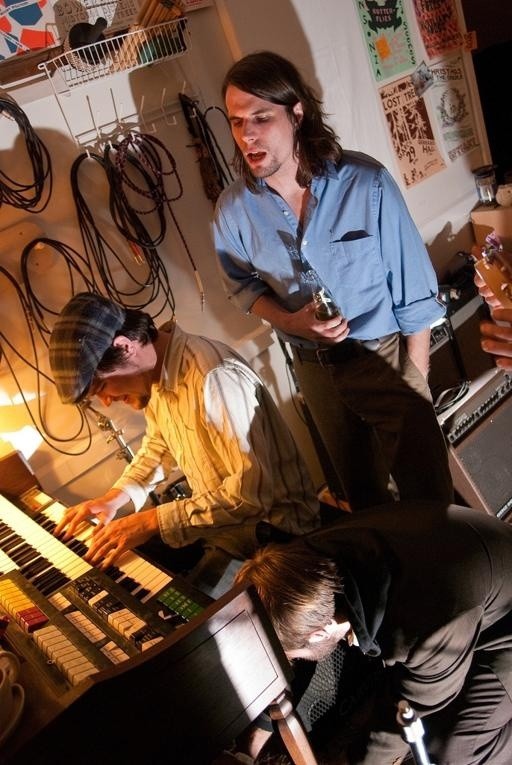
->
[472,164,495,204]
[306,270,339,320]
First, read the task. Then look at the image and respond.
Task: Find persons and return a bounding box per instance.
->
[210,52,451,503]
[468,233,512,373]
[52,289,324,601]
[235,503,512,763]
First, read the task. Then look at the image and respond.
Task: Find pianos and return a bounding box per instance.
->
[0,450,319,765]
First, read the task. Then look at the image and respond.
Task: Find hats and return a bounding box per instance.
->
[48,290,126,405]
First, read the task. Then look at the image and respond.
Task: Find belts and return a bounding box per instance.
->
[295,334,394,368]
